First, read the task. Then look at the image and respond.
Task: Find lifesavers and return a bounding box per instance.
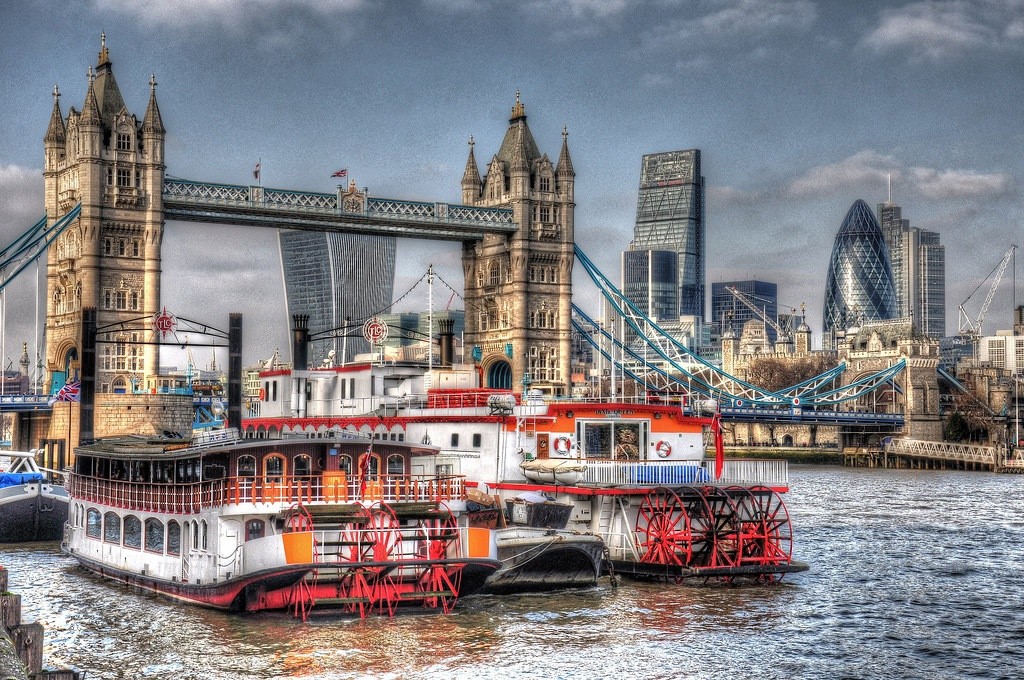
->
[553,436,572,456]
[656,440,672,458]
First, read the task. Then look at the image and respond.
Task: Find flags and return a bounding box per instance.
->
[361,480,367,497]
[48,380,80,408]
[253,163,259,178]
[710,401,725,480]
[360,445,372,479]
[330,169,346,178]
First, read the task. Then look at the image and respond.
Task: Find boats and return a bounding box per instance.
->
[59,427,505,617]
[242,362,807,589]
[0,450,70,540]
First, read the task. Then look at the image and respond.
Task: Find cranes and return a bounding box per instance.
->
[957,244,1017,336]
[724,286,797,340]
